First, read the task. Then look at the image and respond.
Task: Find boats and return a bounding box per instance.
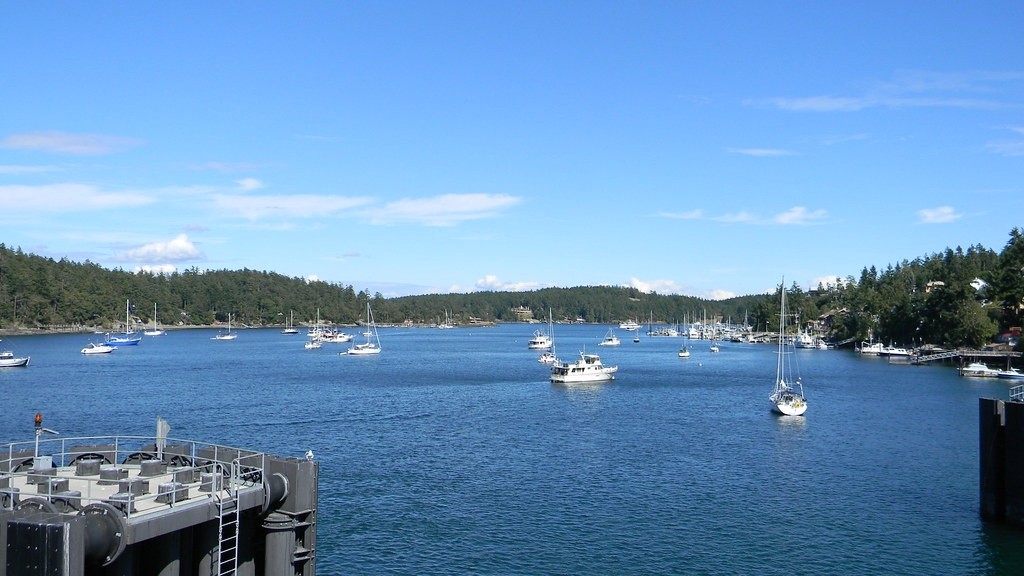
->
[633,339,640,343]
[854,343,883,353]
[549,344,618,383]
[709,347,720,353]
[646,310,836,350]
[619,319,641,328]
[877,345,908,356]
[0,411,317,576]
[598,325,621,345]
[678,351,690,357]
[528,326,552,349]
[626,327,635,331]
[0,356,30,367]
[998,367,1024,379]
[956,363,999,377]
[0,351,14,360]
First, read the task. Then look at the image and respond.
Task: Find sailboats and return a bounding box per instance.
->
[80,299,381,356]
[768,275,807,416]
[537,307,557,363]
